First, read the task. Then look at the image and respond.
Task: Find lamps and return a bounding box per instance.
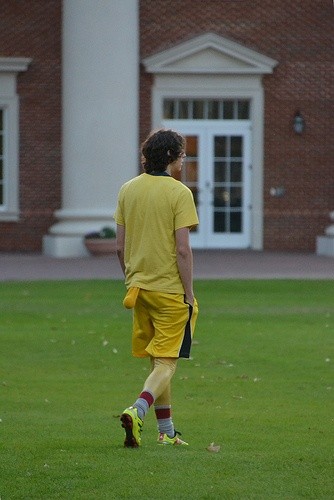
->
[291,109,306,136]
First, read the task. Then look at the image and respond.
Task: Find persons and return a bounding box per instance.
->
[111,128,199,449]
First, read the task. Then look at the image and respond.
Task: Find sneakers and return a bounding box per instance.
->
[157,429,189,446]
[120,406,143,448]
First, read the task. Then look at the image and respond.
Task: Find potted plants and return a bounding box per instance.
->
[85,226,116,255]
